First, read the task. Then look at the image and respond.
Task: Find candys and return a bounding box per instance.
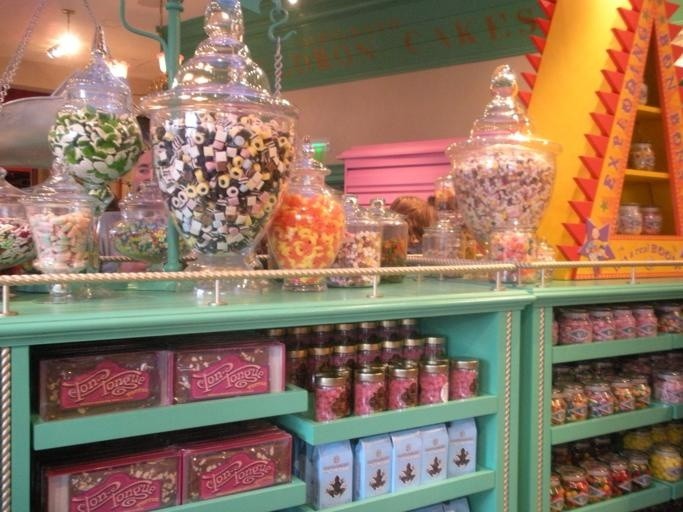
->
[0,104,682,511]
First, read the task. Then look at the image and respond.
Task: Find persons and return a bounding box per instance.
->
[387,194,433,256]
[94,148,155,273]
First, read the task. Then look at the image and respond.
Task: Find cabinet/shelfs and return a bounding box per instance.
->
[614,102,681,238]
[2,285,515,511]
[530,286,683,510]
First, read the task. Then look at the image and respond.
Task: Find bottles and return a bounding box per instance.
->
[0,1,681,303]
[4,302,683,511]
